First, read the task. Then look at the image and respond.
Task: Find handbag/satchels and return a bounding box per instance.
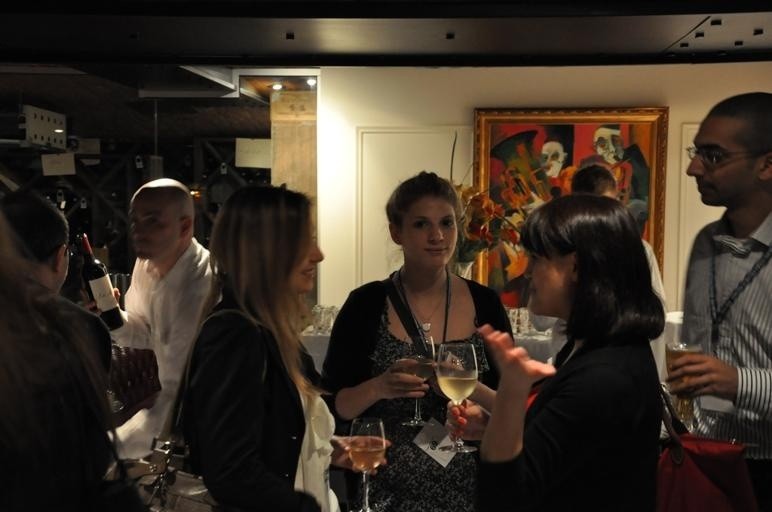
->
[103,435,217,512]
[657,389,757,512]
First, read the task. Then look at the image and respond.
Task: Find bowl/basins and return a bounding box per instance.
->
[529,314,560,332]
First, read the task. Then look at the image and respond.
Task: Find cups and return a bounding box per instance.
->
[311,304,339,335]
[505,306,530,335]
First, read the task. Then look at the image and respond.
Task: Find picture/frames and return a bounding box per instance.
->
[678,121,731,313]
[473,106,671,307]
[357,123,474,295]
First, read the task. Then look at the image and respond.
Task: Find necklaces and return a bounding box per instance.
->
[404,286,446,331]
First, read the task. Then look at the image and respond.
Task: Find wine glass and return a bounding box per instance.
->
[348,417,386,512]
[664,313,709,420]
[400,336,436,428]
[434,343,479,454]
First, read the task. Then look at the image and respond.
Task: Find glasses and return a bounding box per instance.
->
[687,146,753,164]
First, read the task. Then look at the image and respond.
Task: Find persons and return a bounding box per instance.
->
[0,188,70,294]
[321,170,515,512]
[666,91,772,512]
[171,182,392,512]
[530,165,669,382]
[0,209,113,512]
[445,193,665,512]
[80,178,224,496]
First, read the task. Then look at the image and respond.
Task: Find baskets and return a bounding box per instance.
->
[20,105,66,149]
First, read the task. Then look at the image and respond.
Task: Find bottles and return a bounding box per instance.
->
[110,344,158,405]
[75,231,124,333]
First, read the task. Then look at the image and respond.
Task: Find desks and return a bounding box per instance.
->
[512,327,554,364]
[647,310,689,384]
[300,324,329,372]
